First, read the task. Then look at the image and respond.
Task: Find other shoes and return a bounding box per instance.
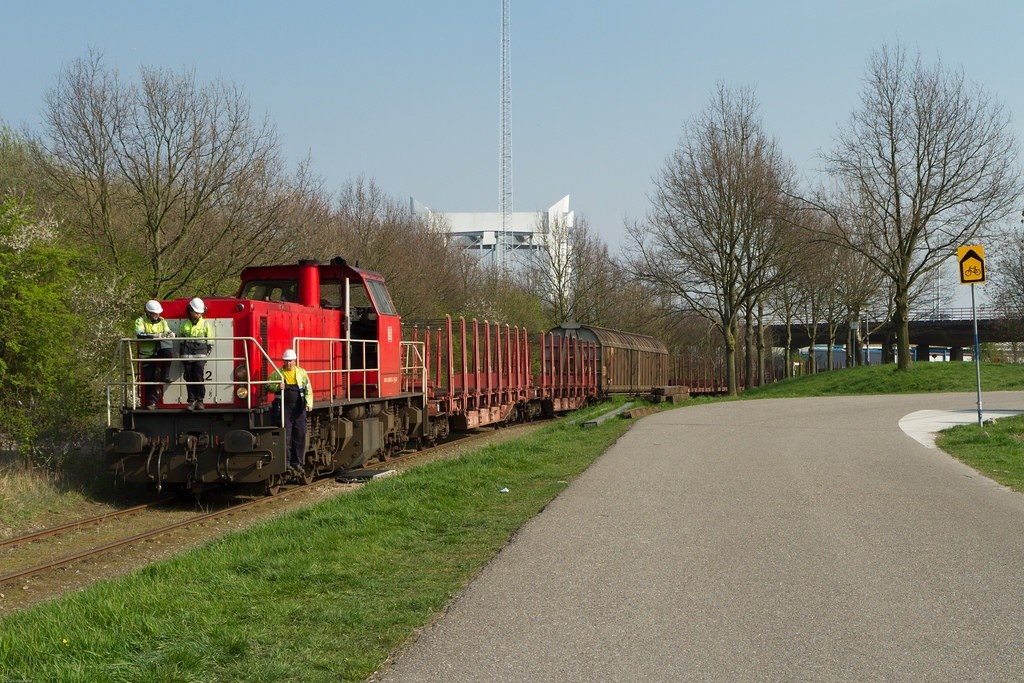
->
[146,402,156,409]
[188,400,196,410]
[286,465,298,476]
[197,400,204,409]
[293,465,305,473]
[160,377,172,383]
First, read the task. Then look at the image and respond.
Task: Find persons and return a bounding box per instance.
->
[180,298,214,410]
[265,349,313,474]
[134,300,177,411]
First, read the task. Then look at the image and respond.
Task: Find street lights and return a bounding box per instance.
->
[861,302,873,366]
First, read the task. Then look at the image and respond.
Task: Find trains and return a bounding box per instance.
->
[103,254,599,500]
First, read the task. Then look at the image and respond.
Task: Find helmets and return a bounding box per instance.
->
[283,349,296,360]
[189,298,204,314]
[146,300,163,314]
